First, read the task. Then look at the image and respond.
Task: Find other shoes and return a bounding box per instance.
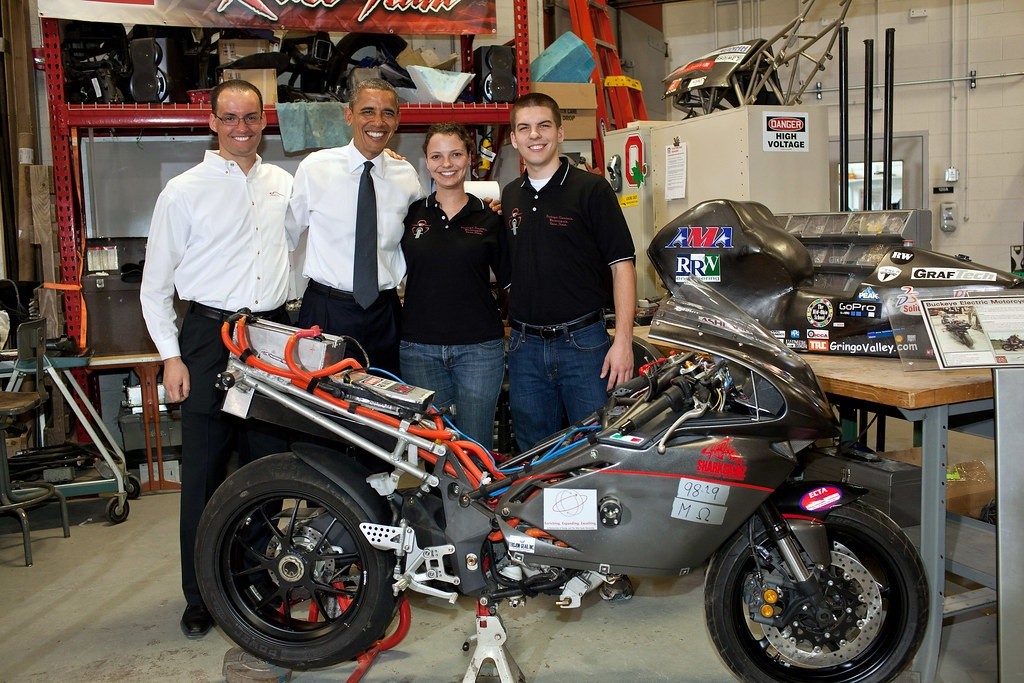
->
[428,580,457,593]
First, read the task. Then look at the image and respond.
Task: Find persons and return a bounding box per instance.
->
[140,80,406,641]
[285,77,501,507]
[402,122,502,476]
[502,92,635,600]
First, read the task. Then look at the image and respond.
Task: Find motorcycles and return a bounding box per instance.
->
[1002,340,1024,351]
[941,314,973,347]
[193,271,932,683]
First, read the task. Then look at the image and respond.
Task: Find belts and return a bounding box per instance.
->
[308,279,397,302]
[510,310,603,338]
[191,305,286,325]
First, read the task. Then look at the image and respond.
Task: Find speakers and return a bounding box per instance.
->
[129,36,174,103]
[474,45,518,105]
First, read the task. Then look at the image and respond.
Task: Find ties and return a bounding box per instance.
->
[353,161,378,310]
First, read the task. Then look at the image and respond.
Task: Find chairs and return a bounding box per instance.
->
[0,318,71,567]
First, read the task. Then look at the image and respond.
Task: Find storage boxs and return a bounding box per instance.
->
[218,39,278,104]
[878,428,996,518]
[528,80,599,141]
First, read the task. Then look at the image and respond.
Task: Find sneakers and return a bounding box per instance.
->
[599,575,634,602]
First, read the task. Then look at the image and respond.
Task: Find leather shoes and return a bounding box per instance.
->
[181,605,213,638]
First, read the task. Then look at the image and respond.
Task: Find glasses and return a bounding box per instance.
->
[215,114,262,125]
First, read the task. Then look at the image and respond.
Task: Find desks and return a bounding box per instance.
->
[40,0,531,473]
[608,330,1019,683]
